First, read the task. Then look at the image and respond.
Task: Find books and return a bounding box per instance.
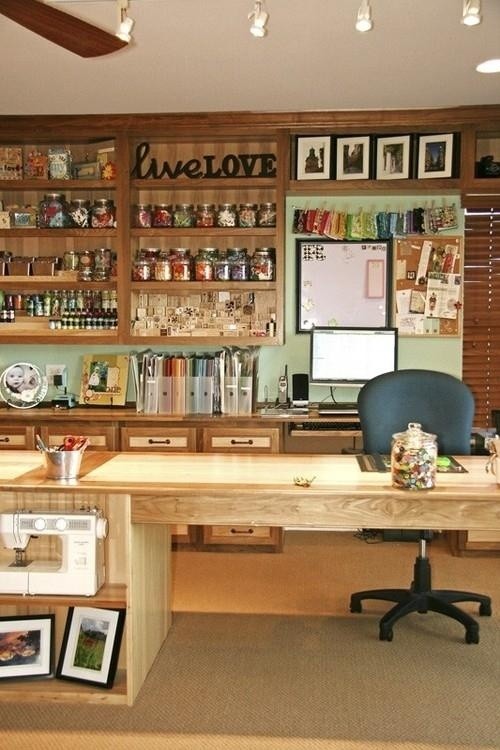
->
[129,347,259,415]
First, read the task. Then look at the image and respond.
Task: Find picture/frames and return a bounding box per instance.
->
[332,134,372,181]
[415,132,456,179]
[0,362,50,410]
[79,354,129,406]
[295,134,332,180]
[373,133,413,180]
[0,612,57,680]
[55,606,127,689]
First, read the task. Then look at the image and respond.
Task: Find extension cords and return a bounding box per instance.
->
[262,409,308,419]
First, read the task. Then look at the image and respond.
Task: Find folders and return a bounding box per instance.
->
[172,354,186,415]
[144,353,158,415]
[158,356,172,415]
[185,356,199,414]
[238,354,260,415]
[223,377,238,415]
[199,355,215,415]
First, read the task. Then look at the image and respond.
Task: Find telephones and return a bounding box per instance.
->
[274,364,291,409]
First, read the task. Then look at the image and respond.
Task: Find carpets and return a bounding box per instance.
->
[0,608,500,750]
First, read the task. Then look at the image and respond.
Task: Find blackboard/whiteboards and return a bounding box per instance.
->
[295,238,394,334]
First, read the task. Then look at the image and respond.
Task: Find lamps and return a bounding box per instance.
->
[115,0,135,42]
[460,1,486,29]
[355,1,373,33]
[246,1,270,39]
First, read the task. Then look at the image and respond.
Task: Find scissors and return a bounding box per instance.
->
[63,435,88,452]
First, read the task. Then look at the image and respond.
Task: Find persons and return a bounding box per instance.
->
[88,366,100,391]
[5,366,24,394]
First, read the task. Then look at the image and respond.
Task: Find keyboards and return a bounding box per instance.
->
[303,422,361,430]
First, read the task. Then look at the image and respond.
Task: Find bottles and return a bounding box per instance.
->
[255,247,276,263]
[76,267,92,282]
[135,203,152,227]
[194,203,217,227]
[94,248,111,266]
[171,247,193,281]
[78,250,95,267]
[93,266,110,283]
[249,252,274,280]
[392,423,438,489]
[173,203,194,227]
[0,288,118,330]
[68,199,91,228]
[259,202,277,228]
[90,199,117,228]
[155,252,172,280]
[227,247,252,281]
[214,252,230,280]
[64,251,78,270]
[218,203,238,227]
[39,194,68,228]
[193,248,219,280]
[133,253,150,280]
[152,203,173,227]
[141,248,161,280]
[239,203,259,227]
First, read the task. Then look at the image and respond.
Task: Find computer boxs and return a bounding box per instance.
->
[383,529,432,542]
[310,326,398,386]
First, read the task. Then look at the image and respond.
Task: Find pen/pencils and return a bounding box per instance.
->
[36,433,48,452]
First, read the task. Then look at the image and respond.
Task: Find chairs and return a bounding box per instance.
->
[347,367,493,645]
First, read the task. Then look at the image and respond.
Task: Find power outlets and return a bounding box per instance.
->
[45,364,67,388]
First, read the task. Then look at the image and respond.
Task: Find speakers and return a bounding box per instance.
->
[293,373,309,406]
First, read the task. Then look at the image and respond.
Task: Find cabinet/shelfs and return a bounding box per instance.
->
[0,105,499,347]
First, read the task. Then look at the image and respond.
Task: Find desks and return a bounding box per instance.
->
[0,405,500,560]
[0,448,499,704]
